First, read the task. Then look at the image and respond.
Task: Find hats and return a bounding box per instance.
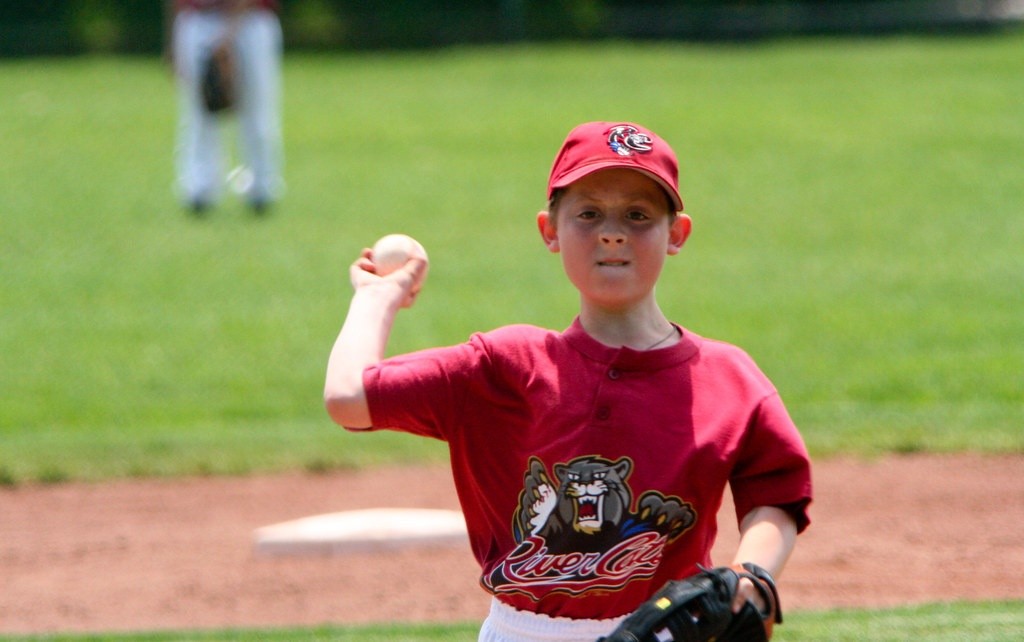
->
[548,121,685,212]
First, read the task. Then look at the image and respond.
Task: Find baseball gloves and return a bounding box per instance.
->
[595,562,783,642]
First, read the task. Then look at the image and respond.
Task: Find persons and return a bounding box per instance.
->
[174,1,289,214]
[323,121,814,642]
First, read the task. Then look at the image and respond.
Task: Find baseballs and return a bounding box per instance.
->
[372,234,428,285]
